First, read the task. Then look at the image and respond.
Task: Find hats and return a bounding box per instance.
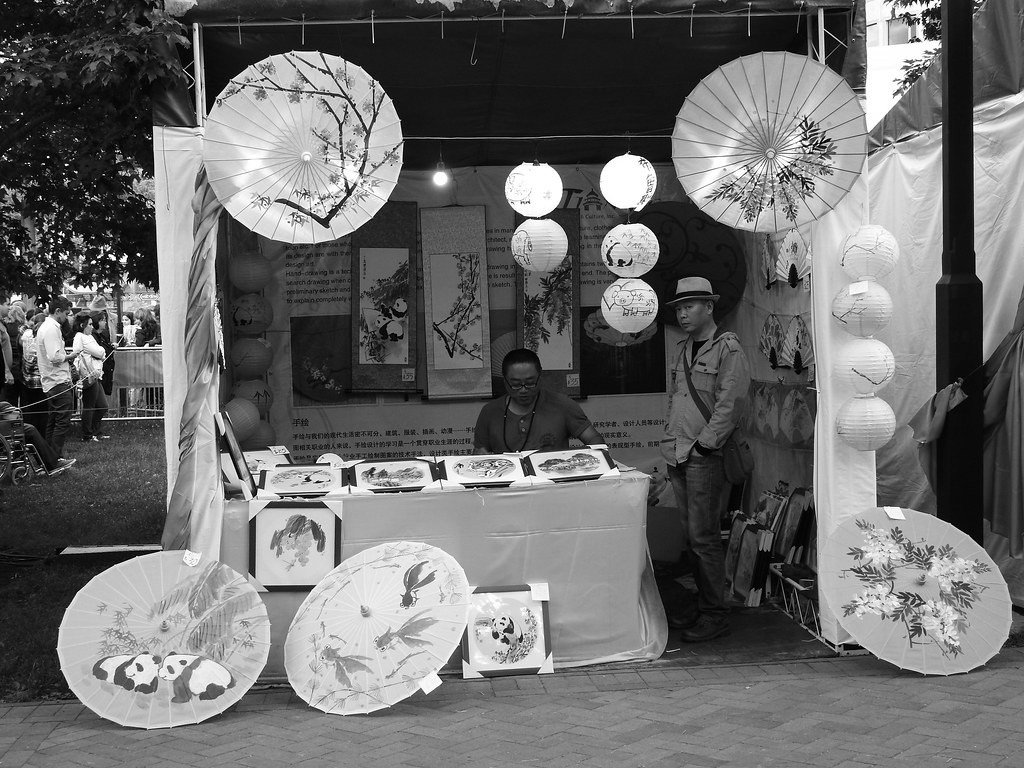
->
[87,295,108,311]
[663,276,721,305]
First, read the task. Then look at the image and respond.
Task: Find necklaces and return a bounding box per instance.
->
[503,390,540,452]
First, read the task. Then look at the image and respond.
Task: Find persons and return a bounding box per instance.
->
[0,289,163,475]
[473,349,604,455]
[664,277,750,642]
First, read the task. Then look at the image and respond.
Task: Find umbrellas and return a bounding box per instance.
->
[55,547,272,730]
[670,51,870,234]
[200,49,405,244]
[822,507,1013,677]
[283,541,471,716]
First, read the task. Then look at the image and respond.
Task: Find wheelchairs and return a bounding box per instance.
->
[0,407,71,487]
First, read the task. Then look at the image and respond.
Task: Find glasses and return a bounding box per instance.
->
[504,375,540,390]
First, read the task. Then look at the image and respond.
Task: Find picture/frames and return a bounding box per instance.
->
[219,444,297,494]
[349,456,442,494]
[521,445,621,484]
[460,583,556,680]
[434,454,532,488]
[247,498,341,592]
[258,459,349,500]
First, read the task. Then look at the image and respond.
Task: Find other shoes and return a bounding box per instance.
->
[47,458,77,476]
[81,434,110,442]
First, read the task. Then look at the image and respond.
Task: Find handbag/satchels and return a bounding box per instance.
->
[721,429,753,483]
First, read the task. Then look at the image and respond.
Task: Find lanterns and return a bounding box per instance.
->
[221,250,276,450]
[584,307,657,346]
[511,218,568,271]
[831,225,901,451]
[601,222,660,279]
[601,279,658,335]
[505,161,563,218]
[599,154,657,209]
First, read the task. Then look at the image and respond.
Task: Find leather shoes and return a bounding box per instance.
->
[680,614,731,645]
[670,611,698,629]
